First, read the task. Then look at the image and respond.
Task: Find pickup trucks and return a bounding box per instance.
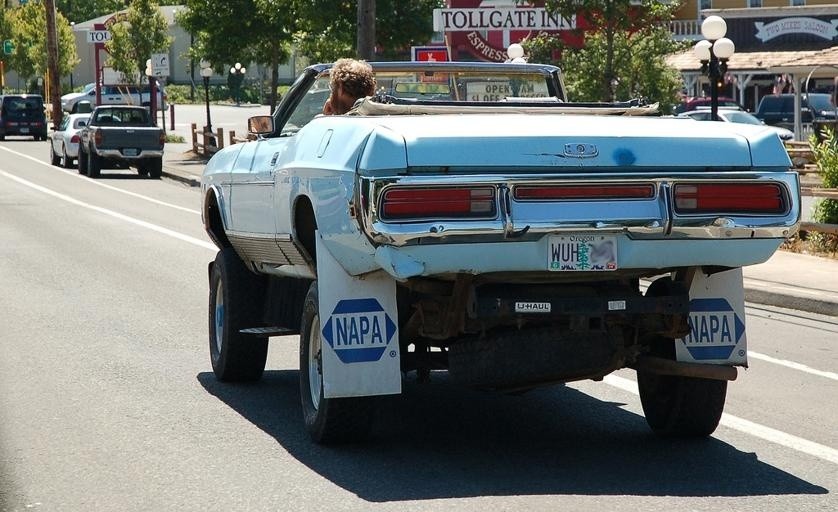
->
[76,103,167,179]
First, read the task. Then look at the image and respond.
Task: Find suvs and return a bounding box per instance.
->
[192,46,811,453]
[61,79,170,116]
[0,92,48,142]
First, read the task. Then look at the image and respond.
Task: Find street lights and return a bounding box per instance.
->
[197,55,216,150]
[229,61,247,106]
[692,14,736,121]
[501,43,527,97]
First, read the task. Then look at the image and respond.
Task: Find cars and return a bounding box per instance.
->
[48,112,124,169]
[672,88,837,143]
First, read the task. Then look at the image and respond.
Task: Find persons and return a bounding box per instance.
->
[323,58,376,116]
